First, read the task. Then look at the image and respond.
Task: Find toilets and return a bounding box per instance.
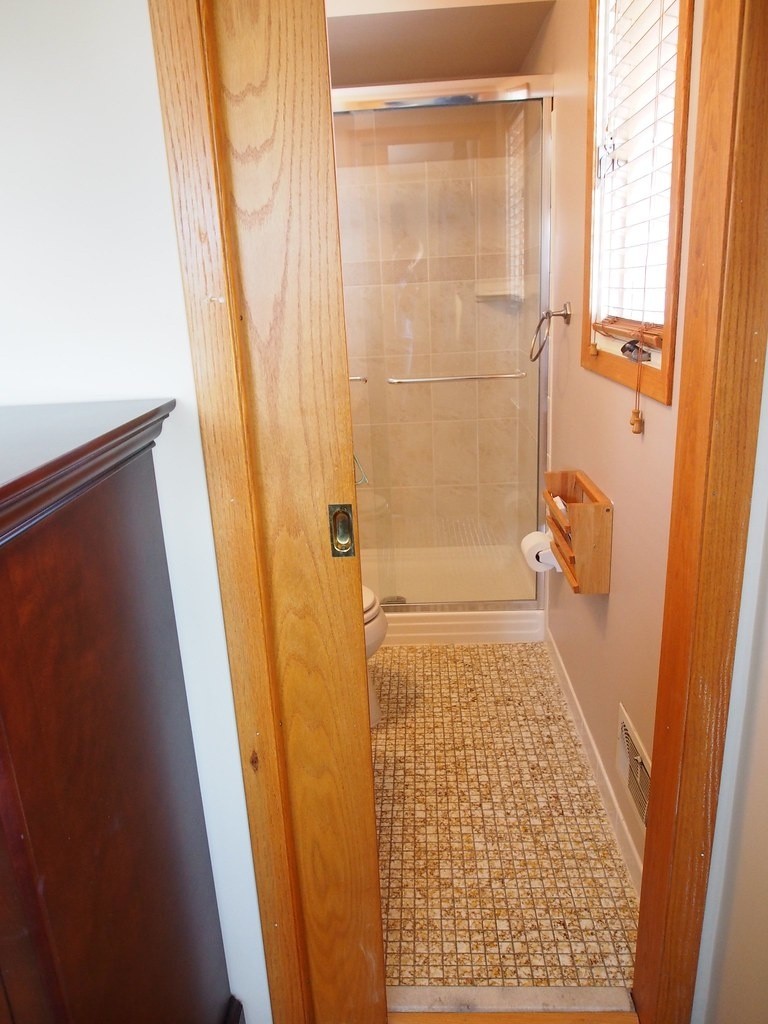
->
[362,584,388,728]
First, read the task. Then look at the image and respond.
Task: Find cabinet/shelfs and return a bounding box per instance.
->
[1,396,249,1024]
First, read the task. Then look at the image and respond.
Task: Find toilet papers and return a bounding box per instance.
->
[520,531,557,572]
